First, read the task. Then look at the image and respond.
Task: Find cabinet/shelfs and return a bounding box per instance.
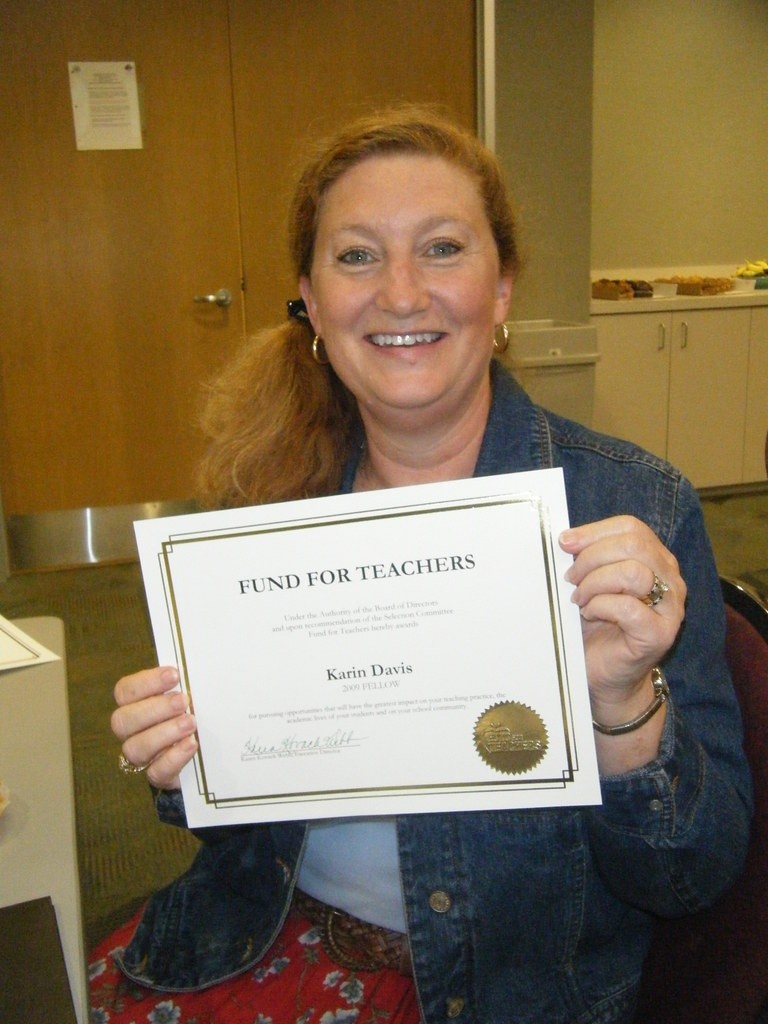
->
[586,305,768,498]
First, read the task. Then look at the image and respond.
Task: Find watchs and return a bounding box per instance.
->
[591,666,669,736]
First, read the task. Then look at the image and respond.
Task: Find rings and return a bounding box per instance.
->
[638,570,670,603]
[117,751,151,774]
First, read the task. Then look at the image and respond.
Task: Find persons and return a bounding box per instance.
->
[111,108,750,1024]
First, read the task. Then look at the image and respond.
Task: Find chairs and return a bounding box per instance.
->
[627,572,768,1024]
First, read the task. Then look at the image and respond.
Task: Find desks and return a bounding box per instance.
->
[0,615,89,1024]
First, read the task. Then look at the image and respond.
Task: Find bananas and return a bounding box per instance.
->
[731,260,768,277]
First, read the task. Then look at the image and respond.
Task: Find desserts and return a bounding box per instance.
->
[592,275,731,301]
